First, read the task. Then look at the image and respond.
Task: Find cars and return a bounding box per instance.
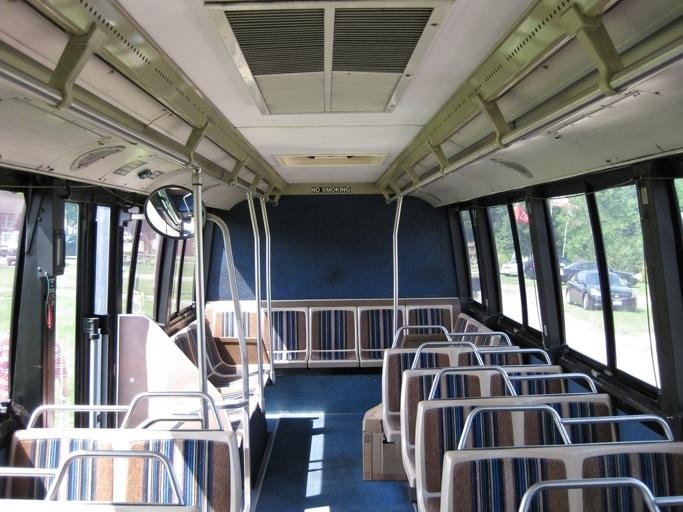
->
[499,256,531,277]
[524,253,573,282]
[558,261,641,288]
[564,270,637,312]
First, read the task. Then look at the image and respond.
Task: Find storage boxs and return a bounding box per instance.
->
[362,403,407,482]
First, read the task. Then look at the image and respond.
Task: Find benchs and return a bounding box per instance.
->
[382,315,683,512]
[211,304,454,376]
[0,390,240,512]
[171,319,274,487]
